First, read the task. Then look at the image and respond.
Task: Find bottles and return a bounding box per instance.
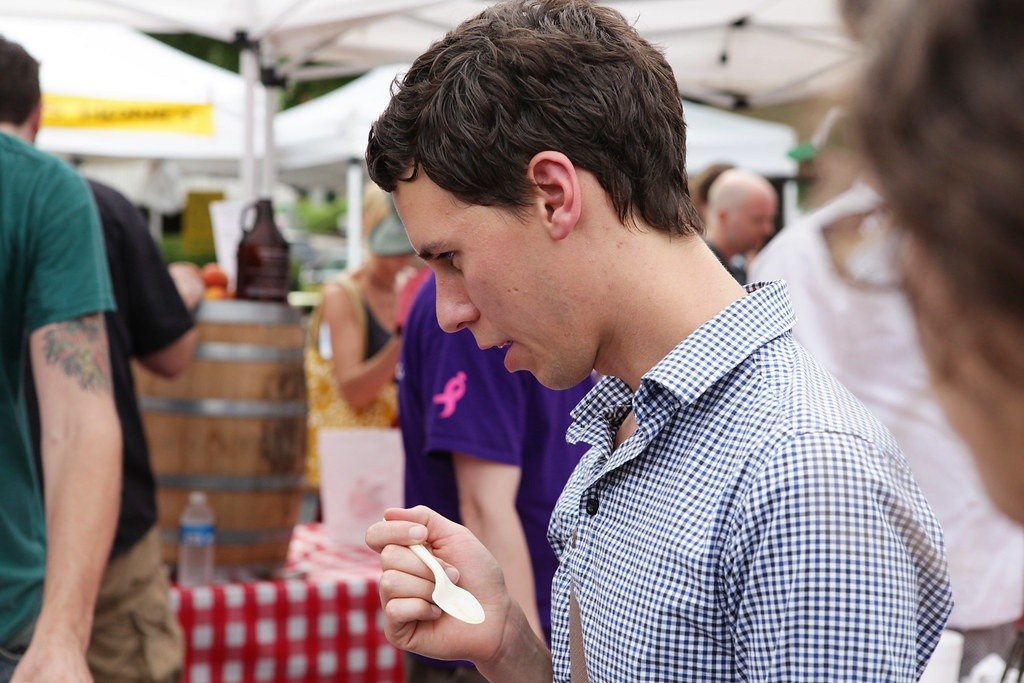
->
[177,492,215,588]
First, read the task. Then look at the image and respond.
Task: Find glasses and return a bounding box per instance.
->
[826,185,887,297]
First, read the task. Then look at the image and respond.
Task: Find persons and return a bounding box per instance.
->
[366,0,955,683]
[305,1,1024,683]
[0,34,206,683]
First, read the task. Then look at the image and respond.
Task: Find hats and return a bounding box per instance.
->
[370,217,411,257]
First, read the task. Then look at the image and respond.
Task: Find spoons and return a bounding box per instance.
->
[383,515,485,624]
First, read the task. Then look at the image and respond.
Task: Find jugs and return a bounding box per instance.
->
[234,202,291,302]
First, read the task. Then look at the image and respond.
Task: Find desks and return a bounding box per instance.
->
[155,524,407,680]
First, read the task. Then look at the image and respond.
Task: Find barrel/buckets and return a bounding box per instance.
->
[121,292,308,587]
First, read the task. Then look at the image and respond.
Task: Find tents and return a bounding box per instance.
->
[0,3,859,286]
